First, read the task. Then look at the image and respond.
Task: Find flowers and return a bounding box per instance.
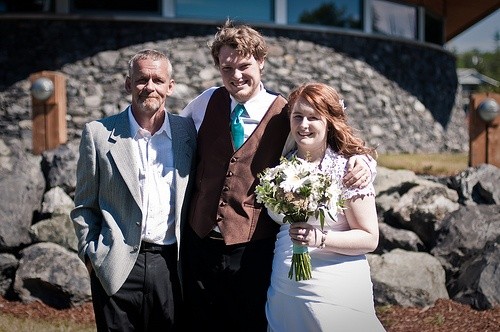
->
[255,151,351,281]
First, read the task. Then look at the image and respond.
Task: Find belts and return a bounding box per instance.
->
[139,240,167,253]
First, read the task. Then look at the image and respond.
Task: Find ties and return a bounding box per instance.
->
[231,103,245,151]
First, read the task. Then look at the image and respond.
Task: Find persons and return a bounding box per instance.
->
[265,81,386,332]
[176,17,378,332]
[70,49,197,332]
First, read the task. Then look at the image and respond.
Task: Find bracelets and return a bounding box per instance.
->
[318,229,327,249]
